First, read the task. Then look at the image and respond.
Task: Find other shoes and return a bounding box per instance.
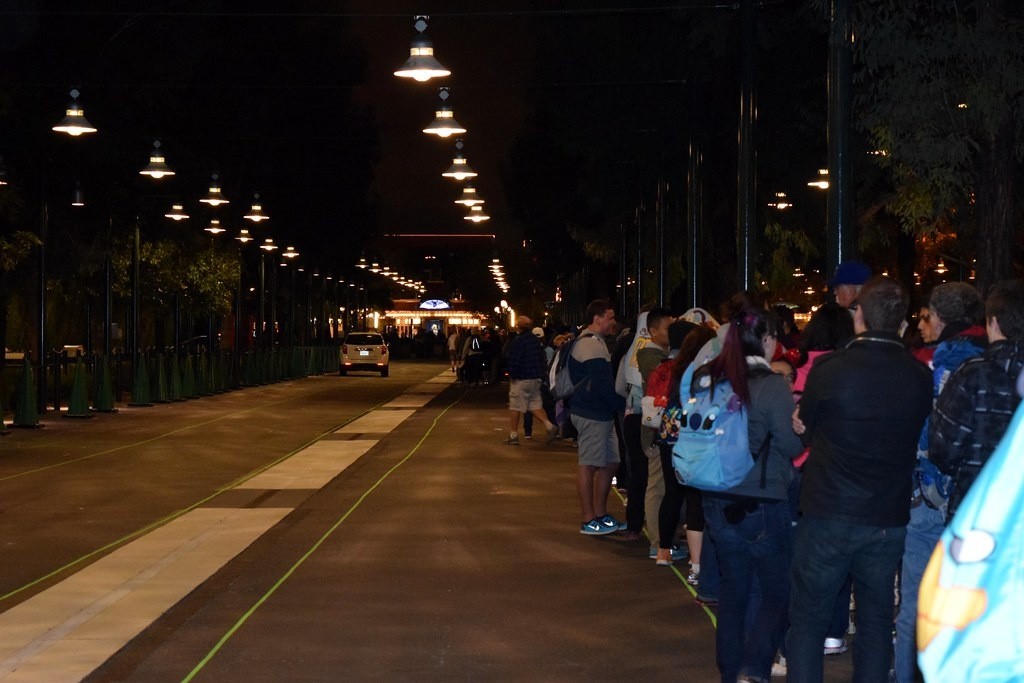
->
[824,637,848,656]
[524,435,532,439]
[561,436,578,448]
[694,594,718,607]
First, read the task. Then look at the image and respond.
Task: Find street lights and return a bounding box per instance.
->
[99,134,368,415]
[28,86,100,414]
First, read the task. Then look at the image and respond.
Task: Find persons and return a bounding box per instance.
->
[448,316,577,446]
[390,326,444,360]
[569,261,1024,683]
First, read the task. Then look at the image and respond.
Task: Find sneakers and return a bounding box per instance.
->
[580,519,619,535]
[686,568,699,586]
[656,546,674,566]
[503,434,519,445]
[601,513,627,531]
[649,544,689,561]
[545,425,560,445]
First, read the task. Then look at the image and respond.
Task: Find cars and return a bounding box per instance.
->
[336,330,390,377]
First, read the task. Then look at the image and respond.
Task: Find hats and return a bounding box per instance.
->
[516,315,531,326]
[824,257,872,286]
[531,327,544,338]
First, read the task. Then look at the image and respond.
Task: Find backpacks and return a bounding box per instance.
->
[670,369,773,495]
[544,333,603,399]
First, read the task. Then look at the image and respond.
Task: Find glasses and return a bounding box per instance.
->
[917,314,930,323]
